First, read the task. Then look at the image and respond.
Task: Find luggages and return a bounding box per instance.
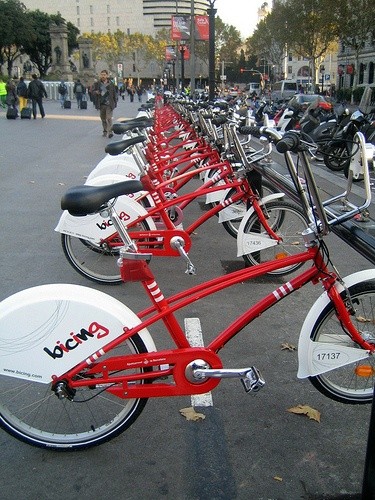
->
[64,95,71,109]
[21,99,31,118]
[80,96,87,109]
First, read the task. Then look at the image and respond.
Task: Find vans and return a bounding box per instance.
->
[270,79,299,103]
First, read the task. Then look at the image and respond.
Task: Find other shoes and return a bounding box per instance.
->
[42,114,45,118]
[7,104,17,119]
[104,132,107,136]
[109,133,113,138]
[34,116,36,119]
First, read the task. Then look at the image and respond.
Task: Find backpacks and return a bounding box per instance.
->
[58,84,66,95]
[76,85,82,92]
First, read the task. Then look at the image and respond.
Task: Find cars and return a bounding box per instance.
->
[286,93,331,114]
[270,90,300,104]
[228,88,241,97]
[240,82,269,99]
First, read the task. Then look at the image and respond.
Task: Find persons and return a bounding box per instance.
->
[0,74,48,119]
[91,70,118,139]
[58,70,142,109]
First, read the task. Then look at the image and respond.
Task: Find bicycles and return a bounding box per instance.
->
[1,125,375,454]
[55,89,375,286]
